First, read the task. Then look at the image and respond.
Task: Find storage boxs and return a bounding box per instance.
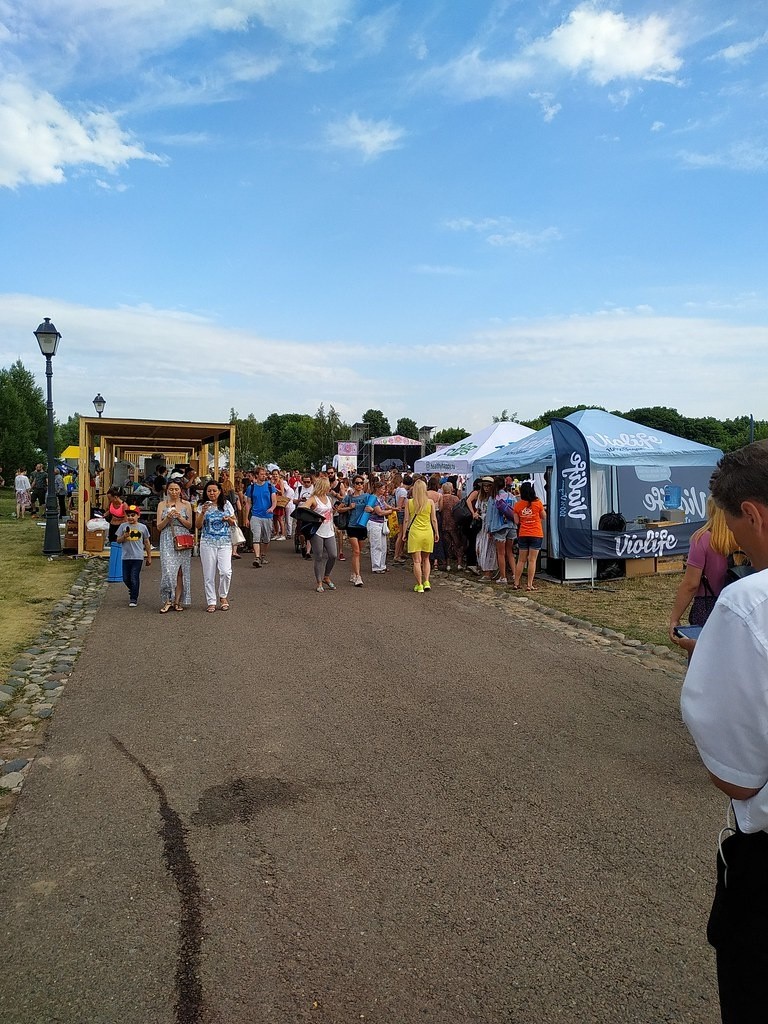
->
[85,526,105,551]
[59,510,78,547]
[625,521,685,578]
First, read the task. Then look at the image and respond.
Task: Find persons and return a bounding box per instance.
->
[672,435,768,1024]
[0,463,553,613]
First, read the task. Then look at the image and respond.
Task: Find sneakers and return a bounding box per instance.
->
[349,573,363,586]
[253,555,268,568]
[129,601,138,607]
[339,553,346,562]
[414,581,431,593]
[271,534,286,541]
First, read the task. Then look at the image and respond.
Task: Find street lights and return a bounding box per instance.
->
[33,317,64,555]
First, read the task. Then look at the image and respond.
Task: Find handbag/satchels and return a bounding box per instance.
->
[172,534,194,551]
[230,521,246,546]
[405,528,410,541]
[383,518,391,536]
[275,490,291,508]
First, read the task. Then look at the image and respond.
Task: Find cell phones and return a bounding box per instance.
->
[302,497,306,499]
[674,624,703,639]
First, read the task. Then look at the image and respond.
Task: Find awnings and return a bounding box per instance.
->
[413,408,725,589]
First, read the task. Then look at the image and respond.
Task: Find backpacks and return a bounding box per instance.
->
[333,494,353,530]
[452,496,472,529]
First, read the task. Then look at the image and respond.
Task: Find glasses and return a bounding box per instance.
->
[327,472,333,475]
[355,482,364,485]
[303,481,310,484]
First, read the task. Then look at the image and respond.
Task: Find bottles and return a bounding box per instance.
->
[638,515,648,524]
[663,484,681,510]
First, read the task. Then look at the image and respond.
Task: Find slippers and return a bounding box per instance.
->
[232,554,241,559]
[316,579,337,593]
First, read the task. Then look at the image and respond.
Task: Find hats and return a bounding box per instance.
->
[170,473,184,480]
[481,476,495,484]
[125,505,140,516]
[241,478,253,485]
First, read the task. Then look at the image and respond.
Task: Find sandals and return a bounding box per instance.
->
[207,598,229,612]
[160,602,183,615]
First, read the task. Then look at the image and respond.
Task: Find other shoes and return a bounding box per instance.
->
[512,583,539,592]
[496,575,516,584]
[394,553,408,564]
[432,564,480,576]
[478,575,495,582]
